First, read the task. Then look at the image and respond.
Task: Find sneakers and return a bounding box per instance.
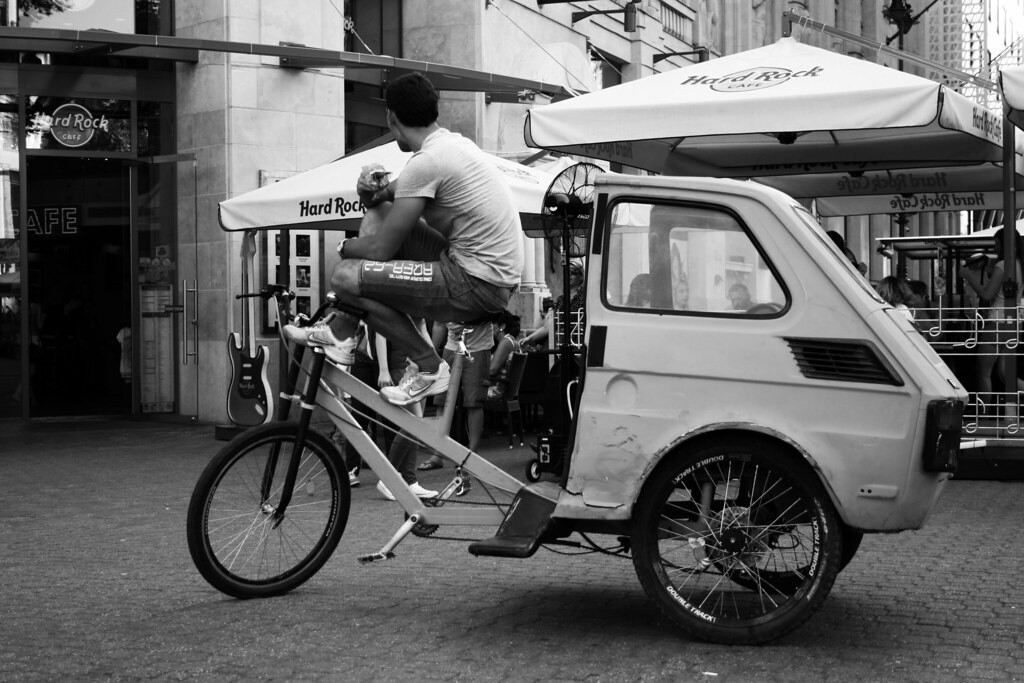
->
[380,357,452,406]
[283,310,357,365]
[409,482,438,497]
[376,480,397,501]
[349,467,360,485]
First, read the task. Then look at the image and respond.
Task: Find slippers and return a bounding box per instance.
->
[417,460,444,470]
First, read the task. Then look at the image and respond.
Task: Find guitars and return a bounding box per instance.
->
[224,229,275,428]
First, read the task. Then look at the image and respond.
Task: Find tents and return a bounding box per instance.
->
[522,36,1024,259]
[217,127,652,238]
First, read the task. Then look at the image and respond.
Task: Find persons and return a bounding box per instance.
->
[490,310,524,397]
[825,228,1024,426]
[626,273,650,307]
[729,283,753,311]
[370,318,438,500]
[283,70,525,405]
[343,324,376,486]
[418,322,494,472]
[518,260,585,402]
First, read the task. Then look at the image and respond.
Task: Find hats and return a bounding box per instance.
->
[569,260,585,276]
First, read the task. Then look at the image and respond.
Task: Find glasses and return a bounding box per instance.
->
[569,270,580,276]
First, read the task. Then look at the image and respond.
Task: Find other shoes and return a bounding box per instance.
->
[965,411,989,421]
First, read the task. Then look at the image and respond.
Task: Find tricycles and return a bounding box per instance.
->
[182,157,970,644]
